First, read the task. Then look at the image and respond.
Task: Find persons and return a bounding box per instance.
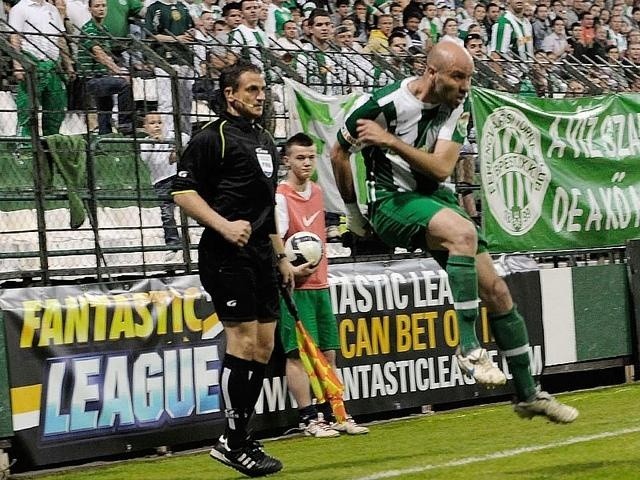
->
[0,1,639,139]
[276,134,370,438]
[168,60,297,477]
[141,111,194,259]
[329,40,581,424]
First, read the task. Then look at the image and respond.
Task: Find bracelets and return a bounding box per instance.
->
[273,250,285,262]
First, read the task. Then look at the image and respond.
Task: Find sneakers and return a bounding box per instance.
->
[208,432,283,477]
[455,345,506,387]
[513,390,579,424]
[300,412,369,437]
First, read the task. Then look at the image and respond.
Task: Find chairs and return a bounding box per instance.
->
[2,137,161,213]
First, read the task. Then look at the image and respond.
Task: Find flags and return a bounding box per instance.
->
[468,88,640,251]
[284,77,372,216]
[294,322,349,426]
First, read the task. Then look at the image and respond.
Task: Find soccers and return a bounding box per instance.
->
[284,230,323,271]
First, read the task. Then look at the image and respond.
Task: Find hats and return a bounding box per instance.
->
[463,33,484,45]
